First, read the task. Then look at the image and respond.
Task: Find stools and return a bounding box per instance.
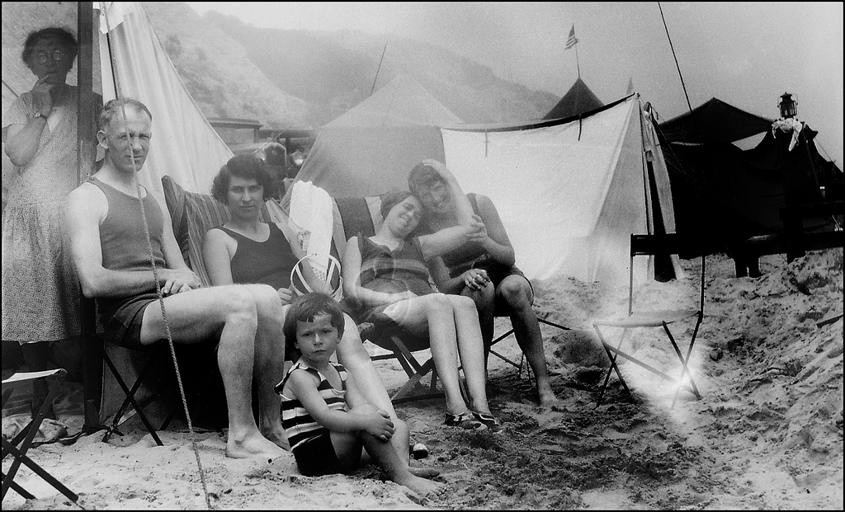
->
[2,371,88,511]
[94,328,176,447]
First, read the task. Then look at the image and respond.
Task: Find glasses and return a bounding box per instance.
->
[36,50,64,65]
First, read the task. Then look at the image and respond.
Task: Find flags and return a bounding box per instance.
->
[561,25,578,53]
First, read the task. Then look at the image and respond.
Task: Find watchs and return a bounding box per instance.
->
[27,111,49,123]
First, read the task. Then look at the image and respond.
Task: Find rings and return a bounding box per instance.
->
[476,274,480,280]
[469,278,475,283]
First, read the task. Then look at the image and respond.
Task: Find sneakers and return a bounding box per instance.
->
[446,410,488,432]
[472,411,500,426]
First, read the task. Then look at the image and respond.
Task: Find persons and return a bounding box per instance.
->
[0,26,103,427]
[273,290,449,500]
[63,98,294,460]
[198,152,400,428]
[339,158,508,440]
[404,161,558,409]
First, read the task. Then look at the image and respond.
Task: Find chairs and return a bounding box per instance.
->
[163,176,269,288]
[331,193,470,400]
[594,234,706,425]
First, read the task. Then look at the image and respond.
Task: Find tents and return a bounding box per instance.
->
[275,72,465,218]
[435,93,685,282]
[646,94,844,276]
[541,79,609,117]
[1,0,239,427]
